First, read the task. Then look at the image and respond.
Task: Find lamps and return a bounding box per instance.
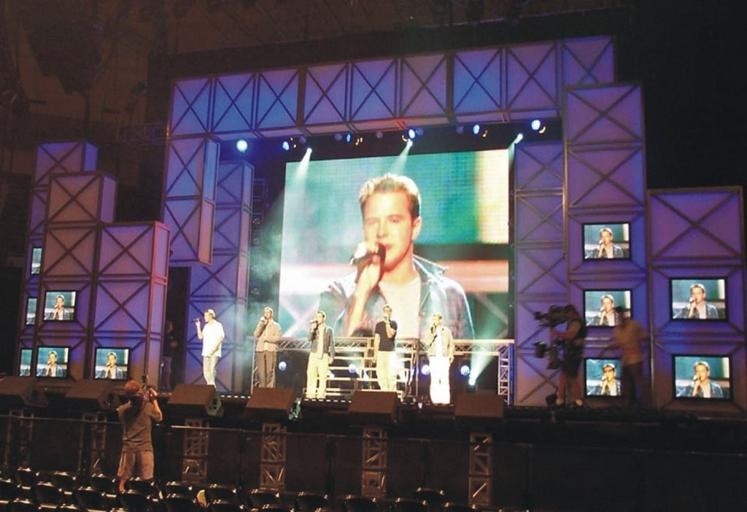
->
[401,128,415,143]
[281,136,299,152]
[531,119,548,135]
[343,131,364,147]
[473,123,489,141]
[237,140,255,158]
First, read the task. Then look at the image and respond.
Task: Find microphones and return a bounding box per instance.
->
[601,375,609,382]
[599,304,607,313]
[692,375,702,382]
[349,243,386,263]
[599,240,606,245]
[311,320,318,324]
[192,319,202,323]
[262,315,267,323]
[689,297,698,304]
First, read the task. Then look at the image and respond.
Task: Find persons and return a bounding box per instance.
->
[40,350,63,377]
[678,285,720,320]
[603,304,651,411]
[195,310,225,390]
[253,306,281,388]
[317,174,474,343]
[117,378,164,490]
[553,306,585,408]
[371,305,398,390]
[590,296,623,327]
[682,361,725,400]
[97,352,123,381]
[589,364,621,398]
[591,227,625,258]
[306,311,335,403]
[424,314,456,407]
[48,295,70,321]
